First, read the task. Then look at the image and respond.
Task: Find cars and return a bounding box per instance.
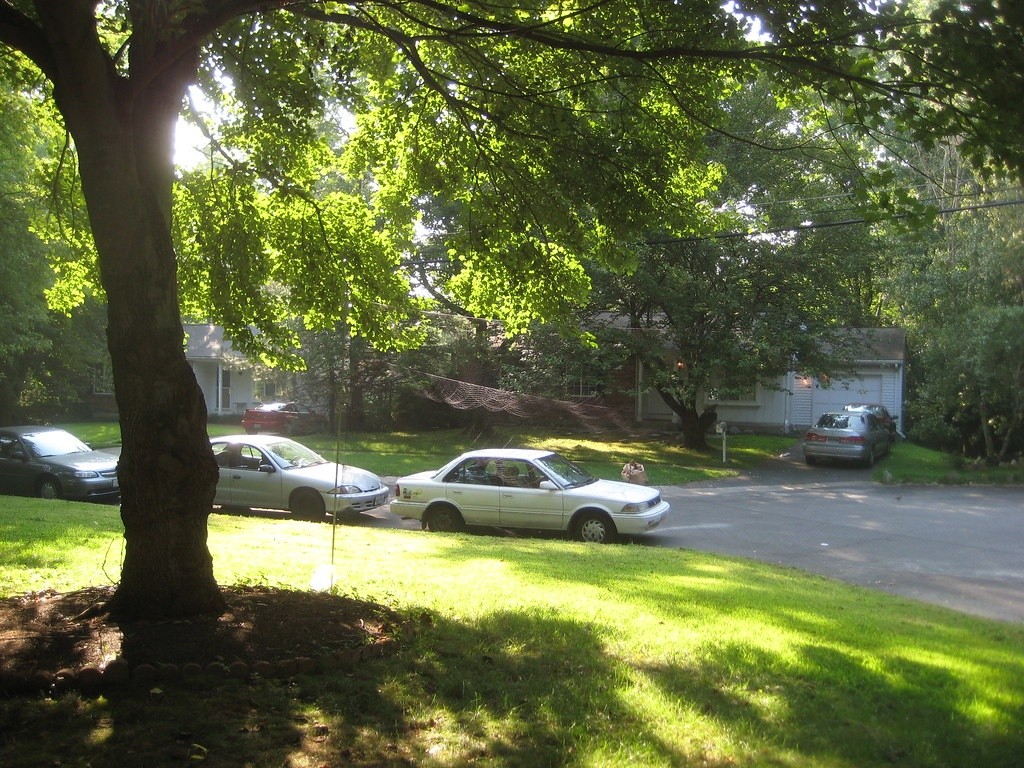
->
[243,402,326,437]
[0,426,120,501]
[802,411,892,470]
[389,449,669,544]
[209,435,389,522]
[843,403,898,440]
